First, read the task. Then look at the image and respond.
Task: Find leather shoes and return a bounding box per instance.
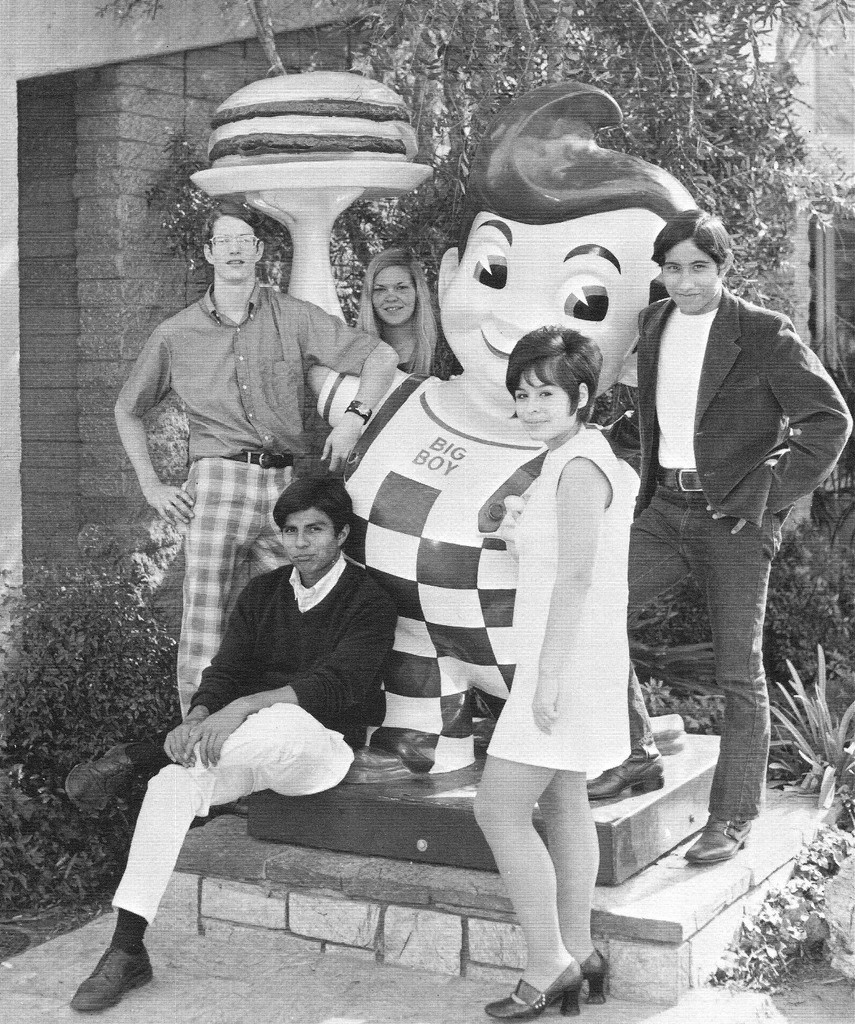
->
[684,815,751,863]
[587,745,665,799]
[65,742,140,809]
[71,948,153,1012]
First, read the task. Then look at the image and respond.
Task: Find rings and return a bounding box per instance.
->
[165,504,174,510]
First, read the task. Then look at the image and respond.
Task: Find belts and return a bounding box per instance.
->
[221,452,294,469]
[663,469,704,492]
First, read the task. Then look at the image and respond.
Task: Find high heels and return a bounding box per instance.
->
[578,949,607,1004]
[485,960,583,1019]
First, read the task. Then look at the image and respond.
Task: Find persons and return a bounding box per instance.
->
[245,82,702,785]
[69,476,397,1013]
[586,209,853,864]
[113,203,400,818]
[356,248,453,382]
[473,326,640,1019]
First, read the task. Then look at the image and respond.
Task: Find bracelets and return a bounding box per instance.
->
[345,400,371,425]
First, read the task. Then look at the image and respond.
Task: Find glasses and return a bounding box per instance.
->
[209,235,260,248]
